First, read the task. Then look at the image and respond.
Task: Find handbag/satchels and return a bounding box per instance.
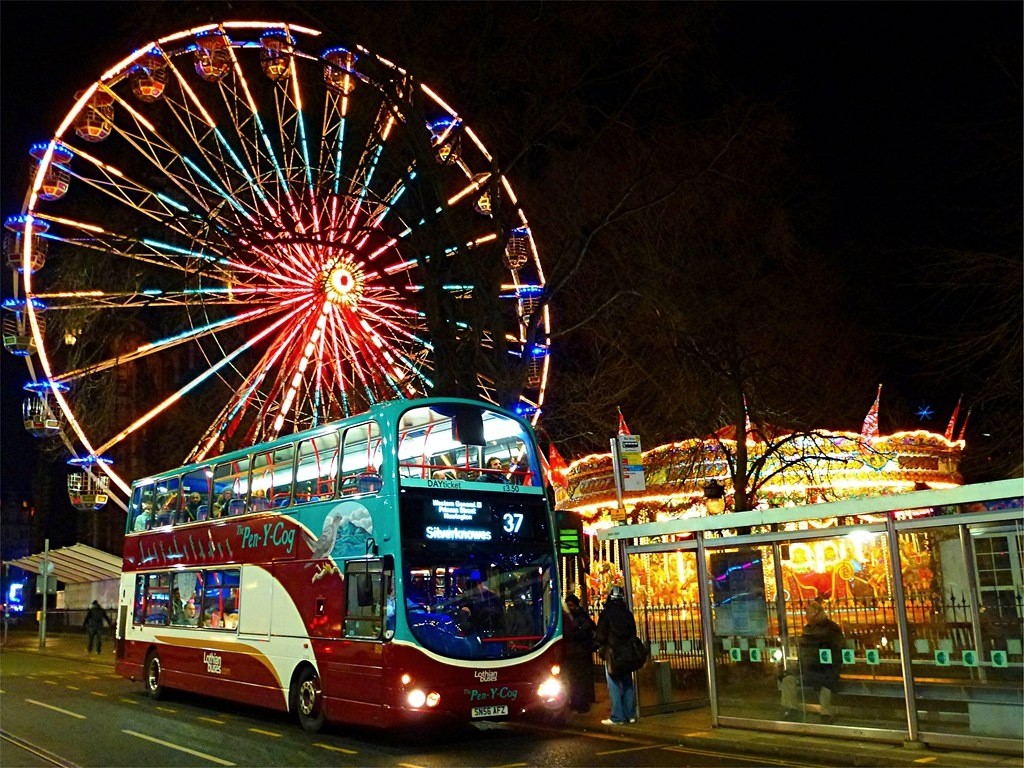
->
[604,633,648,677]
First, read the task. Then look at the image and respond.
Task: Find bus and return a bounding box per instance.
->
[113,398,591,738]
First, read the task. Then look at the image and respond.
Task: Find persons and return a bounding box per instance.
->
[376,569,535,660]
[780,597,843,724]
[562,595,596,712]
[82,599,111,655]
[476,457,511,485]
[592,586,637,725]
[433,471,445,480]
[134,487,266,532]
[166,586,239,629]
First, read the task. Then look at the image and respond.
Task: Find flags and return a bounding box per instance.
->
[549,444,570,488]
[522,448,550,490]
[958,415,970,440]
[744,399,753,442]
[861,393,880,438]
[618,414,632,440]
[945,401,959,441]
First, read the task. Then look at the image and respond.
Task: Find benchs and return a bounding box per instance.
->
[777,672,1023,740]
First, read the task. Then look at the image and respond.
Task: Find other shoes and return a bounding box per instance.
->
[600,718,623,725]
[629,717,636,723]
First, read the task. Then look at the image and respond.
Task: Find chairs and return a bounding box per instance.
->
[133,599,237,630]
[132,471,409,530]
